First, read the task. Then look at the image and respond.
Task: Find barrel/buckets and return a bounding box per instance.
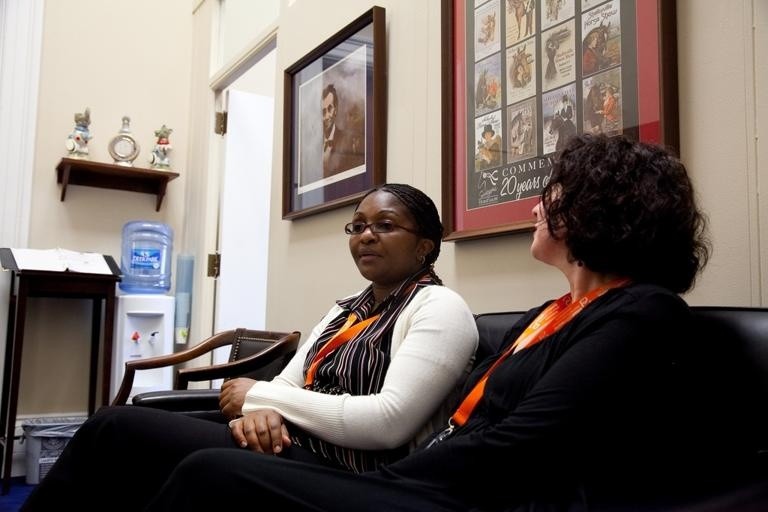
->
[119,220,173,293]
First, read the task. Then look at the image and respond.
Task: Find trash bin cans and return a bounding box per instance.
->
[22,417,83,485]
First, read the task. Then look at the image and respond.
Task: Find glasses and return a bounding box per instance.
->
[343,219,418,234]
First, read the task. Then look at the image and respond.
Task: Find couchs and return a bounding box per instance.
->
[374,306,768,510]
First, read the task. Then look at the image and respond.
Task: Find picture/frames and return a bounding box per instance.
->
[282,6,388,220]
[441,1,680,243]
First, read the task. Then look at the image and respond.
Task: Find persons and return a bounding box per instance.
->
[560,94,573,121]
[145,131,716,512]
[18,182,481,511]
[511,64,529,88]
[594,87,619,125]
[546,40,557,83]
[583,32,598,73]
[320,84,352,177]
[523,0,534,37]
[475,124,501,170]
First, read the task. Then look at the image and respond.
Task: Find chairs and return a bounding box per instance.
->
[115,329,301,422]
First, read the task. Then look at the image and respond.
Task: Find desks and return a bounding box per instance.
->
[0,270,122,498]
